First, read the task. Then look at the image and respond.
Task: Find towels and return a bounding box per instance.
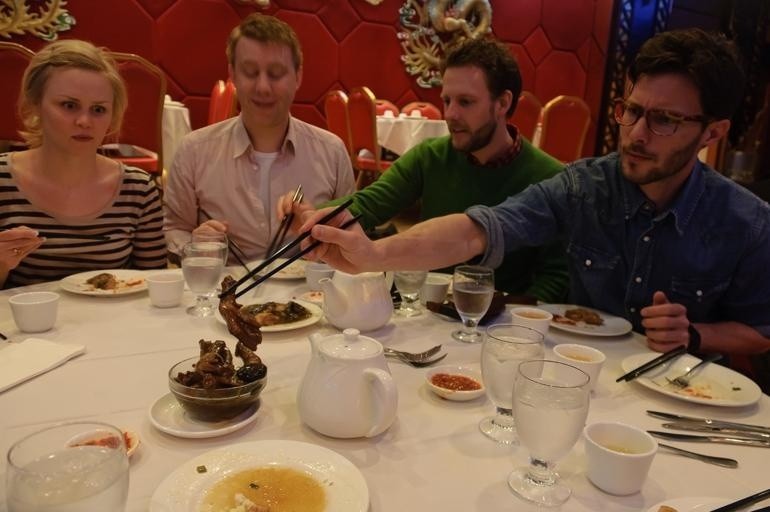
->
[0,339,85,394]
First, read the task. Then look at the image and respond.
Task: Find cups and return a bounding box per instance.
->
[553,344,607,392]
[6,422,129,512]
[8,291,60,334]
[582,421,659,497]
[145,273,185,308]
[421,275,450,308]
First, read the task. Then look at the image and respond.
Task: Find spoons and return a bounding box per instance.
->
[384,353,448,369]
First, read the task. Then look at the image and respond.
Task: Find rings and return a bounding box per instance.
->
[14,248,23,255]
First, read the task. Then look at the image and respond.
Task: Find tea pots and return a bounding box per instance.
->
[296,270,398,440]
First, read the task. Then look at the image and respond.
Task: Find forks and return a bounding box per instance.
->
[384,343,443,362]
[665,355,727,387]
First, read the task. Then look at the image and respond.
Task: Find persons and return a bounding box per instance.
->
[162,14,357,261]
[0,39,167,287]
[276,37,582,304]
[298,38,769,371]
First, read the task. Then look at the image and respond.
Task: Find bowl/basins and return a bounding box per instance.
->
[168,352,268,421]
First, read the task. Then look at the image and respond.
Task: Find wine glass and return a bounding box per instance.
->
[181,230,229,317]
[508,360,588,508]
[452,267,495,342]
[395,266,428,316]
[478,323,545,446]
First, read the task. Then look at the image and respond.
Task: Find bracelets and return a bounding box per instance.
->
[689,322,701,354]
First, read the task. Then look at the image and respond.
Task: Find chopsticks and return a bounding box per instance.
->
[0,227,110,241]
[615,345,687,384]
[197,184,362,300]
[710,490,770,512]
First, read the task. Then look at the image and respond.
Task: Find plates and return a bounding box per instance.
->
[65,389,371,512]
[424,365,488,403]
[622,353,762,407]
[536,304,633,337]
[215,298,323,332]
[59,269,150,297]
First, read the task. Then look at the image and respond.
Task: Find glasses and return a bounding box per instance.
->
[611,97,708,137]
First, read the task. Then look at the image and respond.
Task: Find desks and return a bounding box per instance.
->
[377,117,446,159]
[163,99,193,172]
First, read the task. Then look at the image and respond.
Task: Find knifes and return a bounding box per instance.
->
[645,410,770,448]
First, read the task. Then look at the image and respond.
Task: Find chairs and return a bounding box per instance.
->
[209,77,238,127]
[374,99,397,116]
[349,86,394,185]
[106,51,166,149]
[322,91,351,156]
[531,93,591,165]
[401,102,443,121]
[0,42,39,149]
